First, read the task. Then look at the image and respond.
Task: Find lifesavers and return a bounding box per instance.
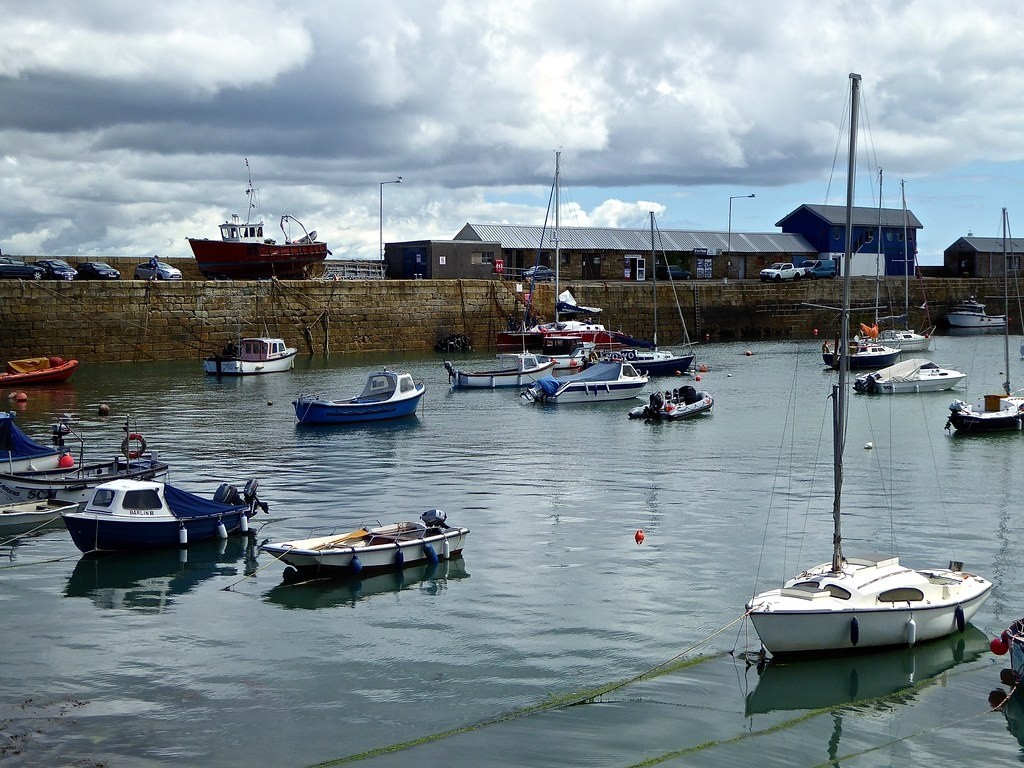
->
[496,263,503,272]
[121,433,147,458]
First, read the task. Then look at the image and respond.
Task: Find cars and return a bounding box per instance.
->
[515,264,556,283]
[134,262,183,280]
[33,258,78,280]
[657,265,692,280]
[75,261,121,280]
[0,256,48,281]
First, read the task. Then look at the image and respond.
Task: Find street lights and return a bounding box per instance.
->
[380,176,403,280]
[727,193,756,279]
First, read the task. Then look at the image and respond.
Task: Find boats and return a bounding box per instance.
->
[0,357,80,388]
[259,508,472,577]
[291,367,430,423]
[1002,619,1024,684]
[58,475,271,554]
[627,385,715,421]
[184,157,333,280]
[0,410,72,474]
[441,143,701,404]
[0,414,171,513]
[0,498,80,537]
[202,311,299,376]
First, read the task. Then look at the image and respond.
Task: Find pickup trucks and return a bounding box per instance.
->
[759,262,806,283]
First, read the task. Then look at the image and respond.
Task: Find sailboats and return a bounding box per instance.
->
[820,165,1024,435]
[745,622,992,768]
[745,74,995,658]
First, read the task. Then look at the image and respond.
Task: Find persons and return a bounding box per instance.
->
[149,255,159,280]
[584,318,593,325]
[822,340,830,353]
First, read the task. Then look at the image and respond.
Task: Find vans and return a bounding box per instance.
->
[799,260,837,280]
